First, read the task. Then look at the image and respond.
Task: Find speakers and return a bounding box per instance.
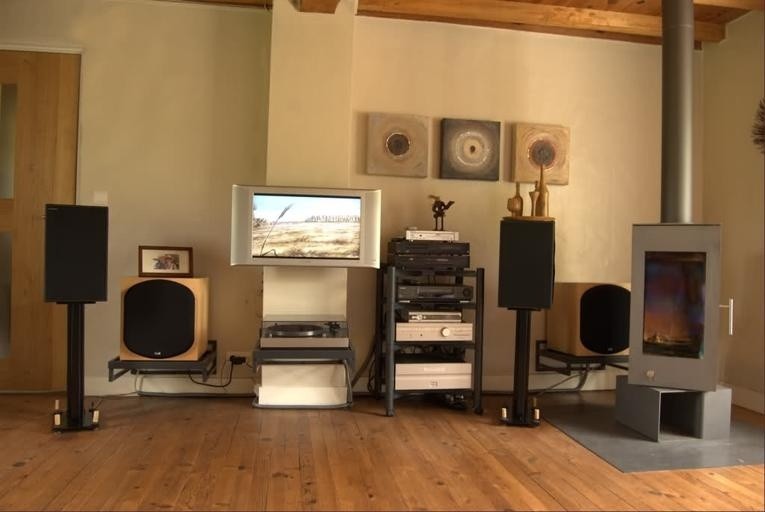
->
[43,204,108,306]
[546,281,631,358]
[497,218,555,313]
[119,278,210,362]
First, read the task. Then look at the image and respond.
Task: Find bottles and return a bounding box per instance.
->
[514,181,523,217]
[536,163,549,217]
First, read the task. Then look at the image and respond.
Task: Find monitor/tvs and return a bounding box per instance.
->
[230,184,381,270]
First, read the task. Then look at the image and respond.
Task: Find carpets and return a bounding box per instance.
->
[533,402,765,473]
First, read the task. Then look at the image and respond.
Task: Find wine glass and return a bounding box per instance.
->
[507,198,520,221]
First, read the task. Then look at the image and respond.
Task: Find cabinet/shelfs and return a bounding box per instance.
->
[373,263,485,417]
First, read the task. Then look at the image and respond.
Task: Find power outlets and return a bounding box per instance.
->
[226,352,253,378]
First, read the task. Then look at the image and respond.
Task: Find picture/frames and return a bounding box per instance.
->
[139,245,193,277]
